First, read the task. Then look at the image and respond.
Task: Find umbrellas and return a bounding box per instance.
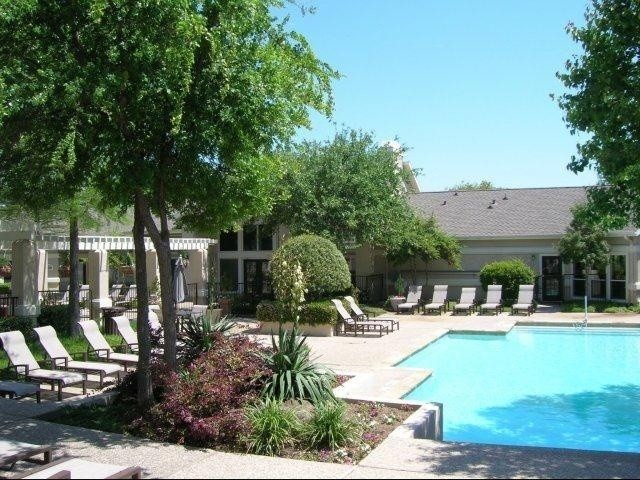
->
[173,254,189,309]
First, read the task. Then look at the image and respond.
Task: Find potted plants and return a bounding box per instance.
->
[390,278,406,311]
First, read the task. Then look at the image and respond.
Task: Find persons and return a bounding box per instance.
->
[58,258,70,297]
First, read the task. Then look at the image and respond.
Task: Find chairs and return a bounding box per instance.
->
[146,302,208,349]
[109,315,180,359]
[0,331,86,402]
[32,325,122,386]
[330,296,399,336]
[0,440,142,480]
[0,363,41,404]
[77,320,157,371]
[396,284,535,318]
[59,283,137,308]
